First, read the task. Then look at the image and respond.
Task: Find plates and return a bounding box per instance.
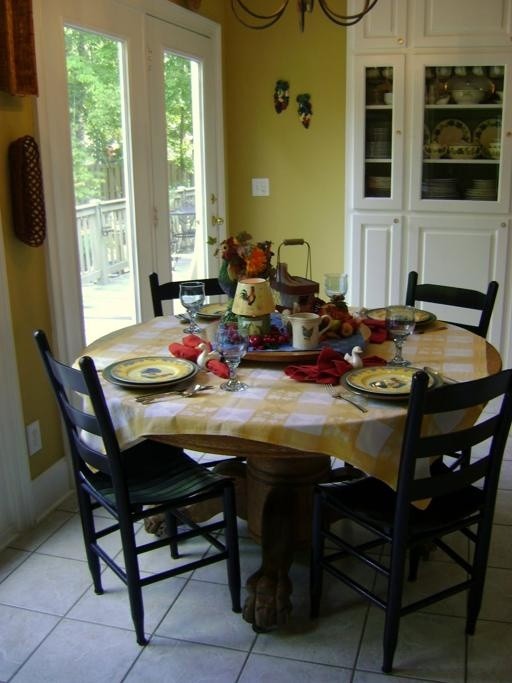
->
[363,305,438,327]
[103,356,199,390]
[364,66,504,197]
[340,366,445,401]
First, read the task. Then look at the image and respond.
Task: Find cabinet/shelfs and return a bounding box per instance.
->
[346,0,512,462]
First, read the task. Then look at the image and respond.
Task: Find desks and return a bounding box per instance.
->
[165,207,197,254]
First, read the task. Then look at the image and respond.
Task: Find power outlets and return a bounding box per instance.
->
[251,178,269,197]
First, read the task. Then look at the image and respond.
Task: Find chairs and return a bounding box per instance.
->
[304,366,512,674]
[150,272,248,474]
[34,330,242,648]
[343,271,499,552]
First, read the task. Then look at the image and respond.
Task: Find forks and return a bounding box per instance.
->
[324,382,368,413]
[136,384,218,405]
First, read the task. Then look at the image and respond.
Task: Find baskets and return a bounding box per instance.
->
[269,238,319,312]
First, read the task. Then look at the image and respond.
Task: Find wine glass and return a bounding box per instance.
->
[175,265,412,392]
